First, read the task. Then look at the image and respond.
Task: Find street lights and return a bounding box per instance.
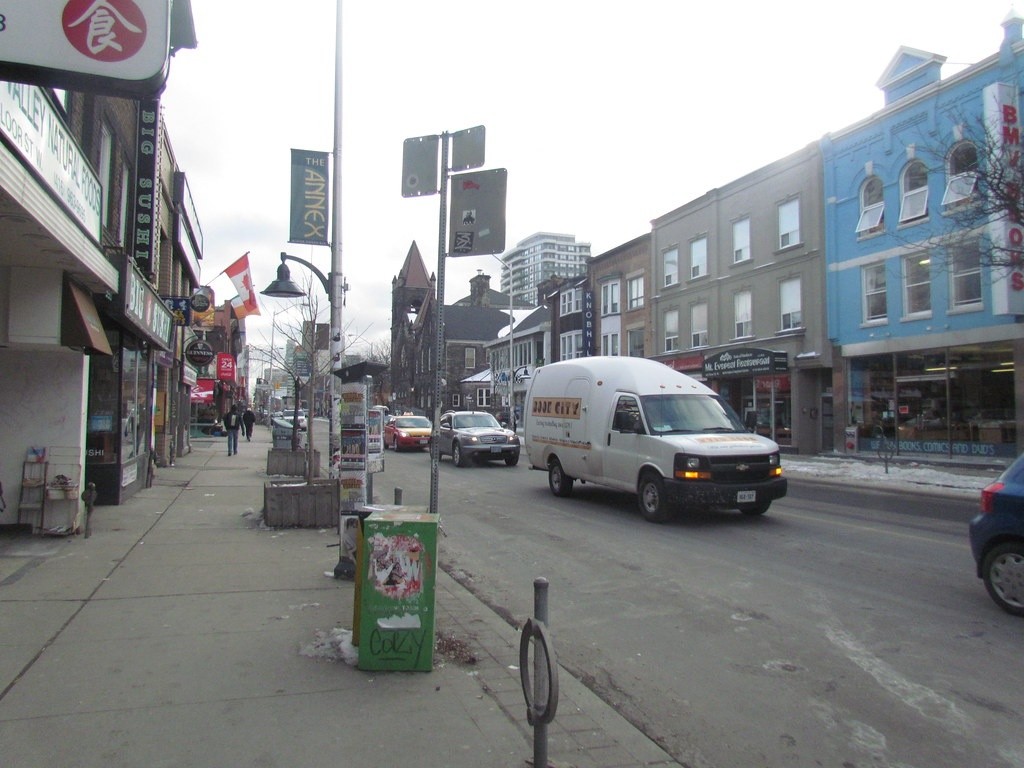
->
[511,365,529,432]
[260,253,344,538]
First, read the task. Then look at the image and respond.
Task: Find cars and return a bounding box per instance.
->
[384,416,434,452]
[283,408,307,432]
[969,452,1024,616]
[429,410,520,467]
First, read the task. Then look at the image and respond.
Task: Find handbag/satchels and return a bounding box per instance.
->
[244,414,255,422]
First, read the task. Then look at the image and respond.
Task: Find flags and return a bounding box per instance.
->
[231,296,262,320]
[224,255,258,312]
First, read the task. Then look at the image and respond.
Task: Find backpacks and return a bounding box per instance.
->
[228,414,239,427]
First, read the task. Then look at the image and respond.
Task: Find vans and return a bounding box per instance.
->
[524,356,788,522]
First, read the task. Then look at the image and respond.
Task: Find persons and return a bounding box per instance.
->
[514,410,519,433]
[225,404,245,456]
[243,408,256,441]
[342,439,365,462]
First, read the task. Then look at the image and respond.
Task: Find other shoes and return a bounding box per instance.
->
[228,453,231,456]
[247,437,250,442]
[234,451,237,455]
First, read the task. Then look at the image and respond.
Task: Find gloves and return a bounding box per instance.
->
[242,432,245,436]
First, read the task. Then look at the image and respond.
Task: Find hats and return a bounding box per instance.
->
[232,404,238,410]
